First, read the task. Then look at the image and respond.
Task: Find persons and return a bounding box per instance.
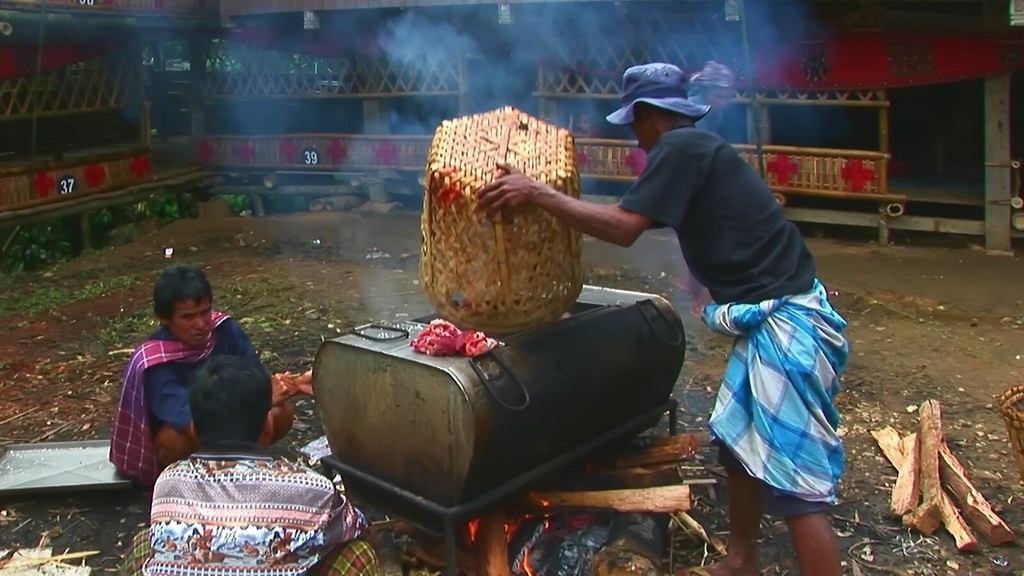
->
[569,113,600,137]
[118,354,381,576]
[110,265,313,486]
[475,62,849,576]
[688,60,747,319]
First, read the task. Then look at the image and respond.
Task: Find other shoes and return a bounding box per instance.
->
[681,565,713,576]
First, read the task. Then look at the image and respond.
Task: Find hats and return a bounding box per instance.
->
[606,62,711,124]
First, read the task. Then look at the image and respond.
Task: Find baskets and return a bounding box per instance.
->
[418,106,581,334]
